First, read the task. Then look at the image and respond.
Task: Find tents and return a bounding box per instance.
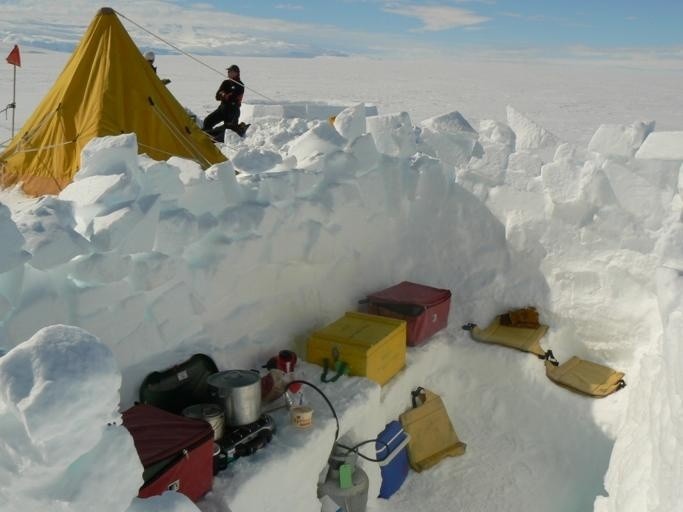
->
[0,7,239,197]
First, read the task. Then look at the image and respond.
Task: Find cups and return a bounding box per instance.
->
[291,406,312,428]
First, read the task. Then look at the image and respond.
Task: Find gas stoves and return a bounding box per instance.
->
[205,413,272,474]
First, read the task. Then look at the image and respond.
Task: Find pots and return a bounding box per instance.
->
[182,368,260,441]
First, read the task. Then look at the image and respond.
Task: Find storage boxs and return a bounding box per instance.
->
[308,312,408,390]
[375,420,412,500]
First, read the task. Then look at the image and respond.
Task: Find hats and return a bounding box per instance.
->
[143,51,155,60]
[226,64,240,71]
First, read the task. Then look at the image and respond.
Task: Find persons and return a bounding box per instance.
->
[203,65,244,129]
[143,51,171,85]
